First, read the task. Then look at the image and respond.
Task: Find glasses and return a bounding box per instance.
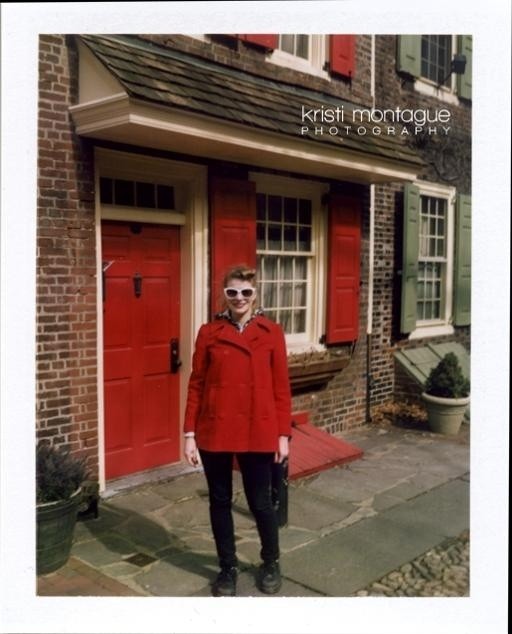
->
[222,285,257,300]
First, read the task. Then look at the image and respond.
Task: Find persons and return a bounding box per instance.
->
[182,262,293,596]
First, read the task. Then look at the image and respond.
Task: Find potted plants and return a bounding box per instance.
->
[36,444,91,576]
[422,351,471,436]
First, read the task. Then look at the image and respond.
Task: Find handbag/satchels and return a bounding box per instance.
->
[271,460,290,530]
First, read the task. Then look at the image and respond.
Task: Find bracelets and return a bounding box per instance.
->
[184,431,196,439]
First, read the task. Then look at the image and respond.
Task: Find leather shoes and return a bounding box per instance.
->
[258,558,284,594]
[213,565,237,596]
